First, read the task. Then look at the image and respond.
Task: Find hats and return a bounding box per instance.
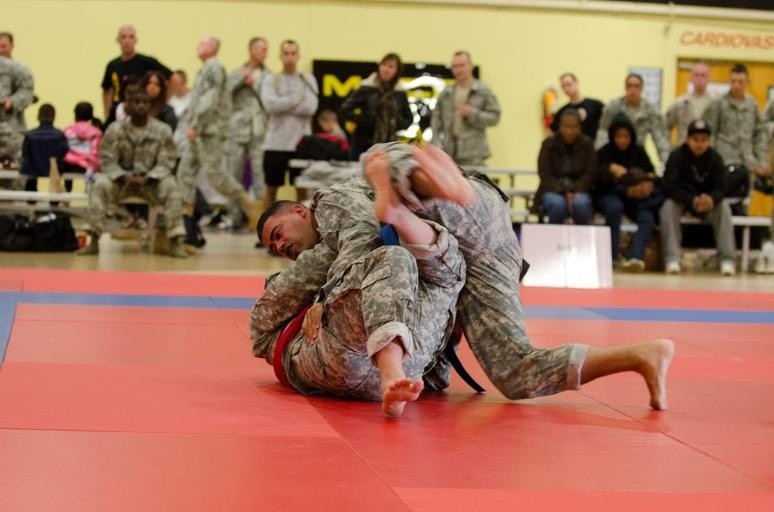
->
[688,119,711,136]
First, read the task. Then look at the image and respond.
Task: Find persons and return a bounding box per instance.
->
[0,25,320,258]
[428,51,500,167]
[247,150,468,417]
[531,61,774,273]
[255,138,674,410]
[320,109,347,140]
[341,53,414,161]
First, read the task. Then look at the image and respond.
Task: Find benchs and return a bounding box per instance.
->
[286,152,772,276]
[0,164,179,254]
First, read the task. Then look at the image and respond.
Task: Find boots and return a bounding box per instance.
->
[239,193,264,230]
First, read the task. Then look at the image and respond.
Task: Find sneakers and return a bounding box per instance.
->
[621,257,645,272]
[666,262,681,273]
[720,260,735,275]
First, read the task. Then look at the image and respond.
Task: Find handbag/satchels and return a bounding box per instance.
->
[726,163,750,216]
[296,132,349,160]
